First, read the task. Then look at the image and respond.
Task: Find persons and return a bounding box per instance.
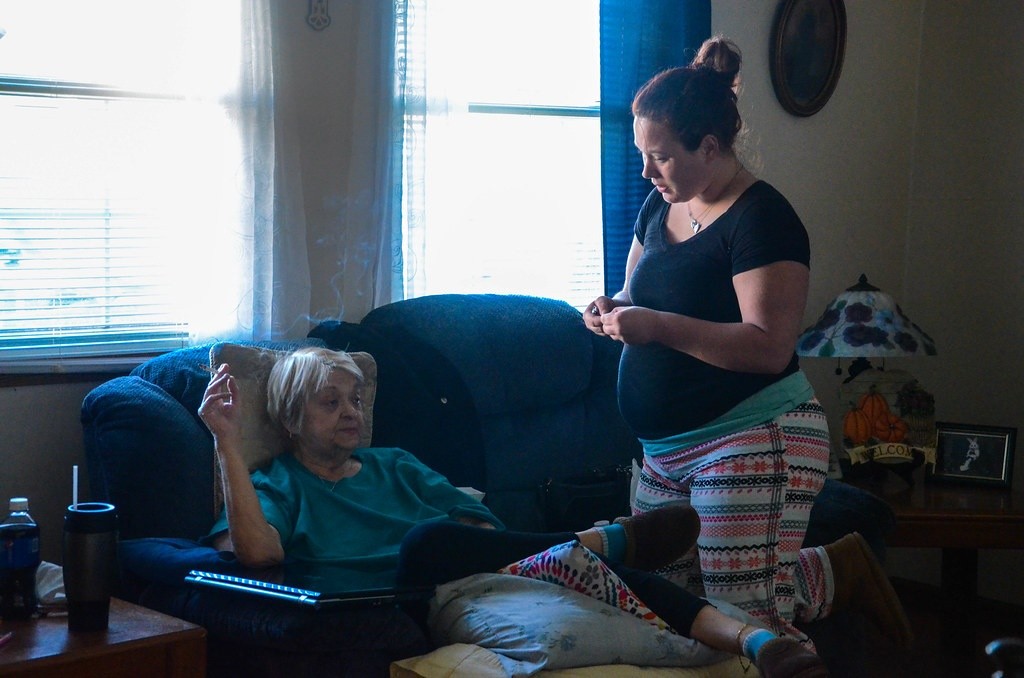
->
[198,347,828,678]
[583,35,914,678]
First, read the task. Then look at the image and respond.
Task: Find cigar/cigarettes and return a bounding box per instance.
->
[199,363,217,374]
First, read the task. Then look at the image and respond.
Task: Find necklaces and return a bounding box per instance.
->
[314,464,353,491]
[687,163,745,232]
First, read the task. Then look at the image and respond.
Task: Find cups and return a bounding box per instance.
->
[63,503,117,632]
[981,638,1024,678]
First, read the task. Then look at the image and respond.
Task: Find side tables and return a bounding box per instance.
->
[0,590,209,678]
[847,474,1024,654]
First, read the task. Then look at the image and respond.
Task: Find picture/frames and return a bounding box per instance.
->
[768,0,847,117]
[931,420,1018,490]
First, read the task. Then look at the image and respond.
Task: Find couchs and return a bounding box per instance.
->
[80,293,896,678]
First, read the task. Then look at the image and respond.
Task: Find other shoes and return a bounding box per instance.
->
[821,531,912,649]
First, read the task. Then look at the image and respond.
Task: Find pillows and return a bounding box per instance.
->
[494,539,677,634]
[208,342,379,522]
[422,570,778,678]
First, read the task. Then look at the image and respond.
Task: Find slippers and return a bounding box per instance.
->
[612,504,701,571]
[752,637,830,678]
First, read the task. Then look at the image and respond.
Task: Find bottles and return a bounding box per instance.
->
[0,498,40,622]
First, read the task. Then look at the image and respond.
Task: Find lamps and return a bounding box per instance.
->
[795,272,938,489]
